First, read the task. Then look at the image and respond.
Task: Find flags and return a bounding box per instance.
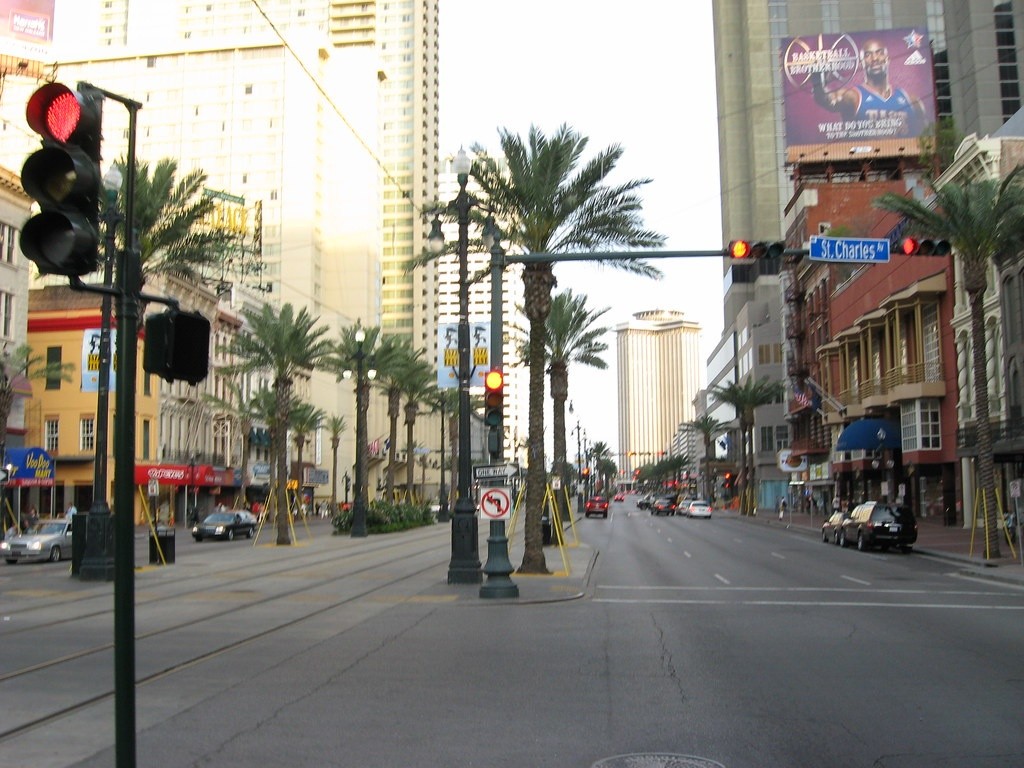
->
[808,382,822,412]
[793,387,810,407]
[369,439,379,455]
[385,438,391,451]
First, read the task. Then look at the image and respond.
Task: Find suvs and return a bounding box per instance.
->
[585,496,609,518]
[840,500,918,554]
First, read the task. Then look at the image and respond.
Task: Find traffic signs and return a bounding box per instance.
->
[473,461,521,481]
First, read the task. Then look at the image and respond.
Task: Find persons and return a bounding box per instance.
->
[189,504,199,527]
[1003,505,1017,546]
[215,502,227,512]
[315,499,330,519]
[776,493,828,515]
[252,501,265,523]
[3,507,39,542]
[64,503,77,520]
[831,494,855,513]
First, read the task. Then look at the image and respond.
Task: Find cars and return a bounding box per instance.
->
[191,510,259,542]
[636,492,712,519]
[0,519,75,563]
[821,510,852,545]
[614,494,625,501]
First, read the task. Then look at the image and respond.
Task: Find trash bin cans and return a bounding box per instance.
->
[149,526,175,563]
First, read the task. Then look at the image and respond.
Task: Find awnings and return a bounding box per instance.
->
[837,417,901,451]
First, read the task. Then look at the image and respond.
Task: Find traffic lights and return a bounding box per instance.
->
[725,237,784,260]
[144,309,212,386]
[900,238,950,259]
[484,371,504,426]
[19,81,105,275]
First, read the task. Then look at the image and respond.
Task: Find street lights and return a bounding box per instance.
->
[426,171,502,583]
[342,329,377,539]
